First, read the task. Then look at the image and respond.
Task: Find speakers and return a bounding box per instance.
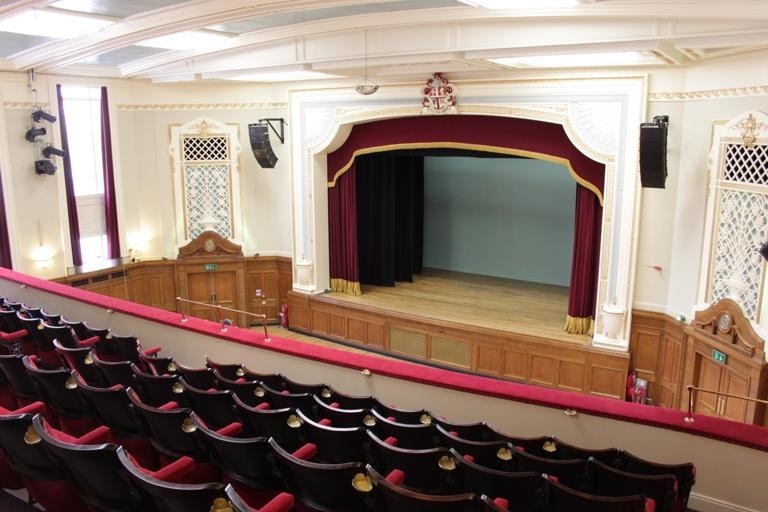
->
[248,124,278,168]
[639,122,668,188]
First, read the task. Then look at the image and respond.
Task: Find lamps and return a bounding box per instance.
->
[35,159,58,176]
[32,108,57,123]
[42,146,65,158]
[24,127,46,142]
[354,30,380,95]
